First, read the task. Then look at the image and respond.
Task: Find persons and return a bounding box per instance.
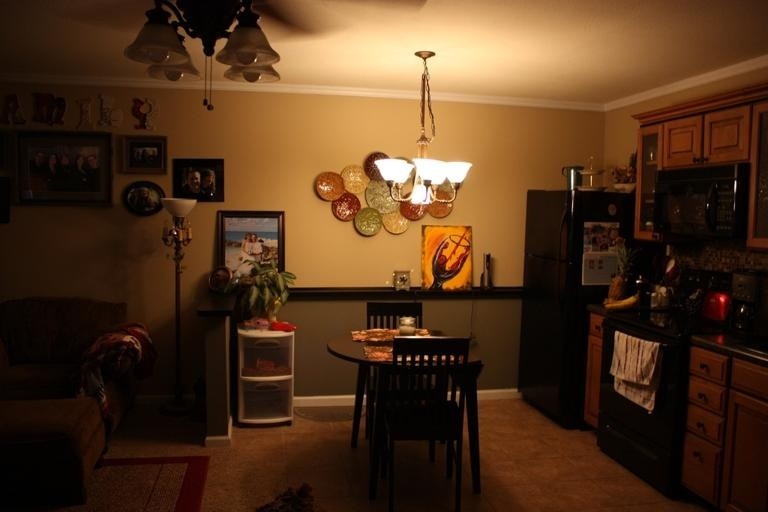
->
[240,233,256,264]
[248,234,263,263]
[173,170,212,202]
[182,169,216,196]
[28,151,103,192]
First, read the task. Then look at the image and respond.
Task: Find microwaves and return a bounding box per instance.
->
[652,163,749,240]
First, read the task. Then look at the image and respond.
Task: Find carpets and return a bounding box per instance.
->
[60,454,211,512]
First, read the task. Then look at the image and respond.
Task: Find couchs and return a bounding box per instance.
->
[0,295,146,436]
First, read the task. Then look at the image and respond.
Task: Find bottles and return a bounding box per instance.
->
[660,245,674,281]
[635,277,672,309]
[479,252,492,289]
[399,316,417,336]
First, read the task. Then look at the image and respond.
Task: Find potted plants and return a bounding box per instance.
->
[226,258,295,325]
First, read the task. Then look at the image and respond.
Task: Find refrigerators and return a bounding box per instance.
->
[513,188,634,429]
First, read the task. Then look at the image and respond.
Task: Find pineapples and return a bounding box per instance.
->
[607,247,633,298]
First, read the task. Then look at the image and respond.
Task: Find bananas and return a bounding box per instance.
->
[604,291,640,309]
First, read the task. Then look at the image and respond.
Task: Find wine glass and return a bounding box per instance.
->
[431,235,472,289]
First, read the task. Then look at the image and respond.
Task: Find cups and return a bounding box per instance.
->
[30,93,65,124]
[561,166,582,191]
[581,154,603,188]
[416,329,431,336]
[393,270,412,292]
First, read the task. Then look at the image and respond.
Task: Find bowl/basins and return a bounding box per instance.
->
[612,183,636,193]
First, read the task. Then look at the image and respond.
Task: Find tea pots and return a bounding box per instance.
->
[701,286,732,325]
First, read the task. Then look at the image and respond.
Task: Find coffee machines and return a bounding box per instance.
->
[731,270,767,334]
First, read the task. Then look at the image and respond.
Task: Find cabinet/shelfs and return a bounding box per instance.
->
[718,355,766,512]
[632,123,660,243]
[235,328,295,427]
[660,103,750,169]
[744,97,766,250]
[679,345,730,506]
[582,312,606,429]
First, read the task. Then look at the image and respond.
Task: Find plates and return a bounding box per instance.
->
[315,152,454,237]
[576,185,607,192]
[364,336,393,343]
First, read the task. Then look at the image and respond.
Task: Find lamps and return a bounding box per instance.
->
[157,195,198,415]
[370,49,474,207]
[120,0,284,112]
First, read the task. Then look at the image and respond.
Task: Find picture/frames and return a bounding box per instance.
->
[171,156,226,203]
[14,127,114,208]
[120,132,167,174]
[213,207,288,284]
[120,180,164,216]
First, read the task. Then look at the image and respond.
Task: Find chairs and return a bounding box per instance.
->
[366,335,470,512]
[347,301,438,464]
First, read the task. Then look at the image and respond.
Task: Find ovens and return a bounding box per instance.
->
[597,317,684,499]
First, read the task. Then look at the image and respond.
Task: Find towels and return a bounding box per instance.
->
[609,328,662,415]
